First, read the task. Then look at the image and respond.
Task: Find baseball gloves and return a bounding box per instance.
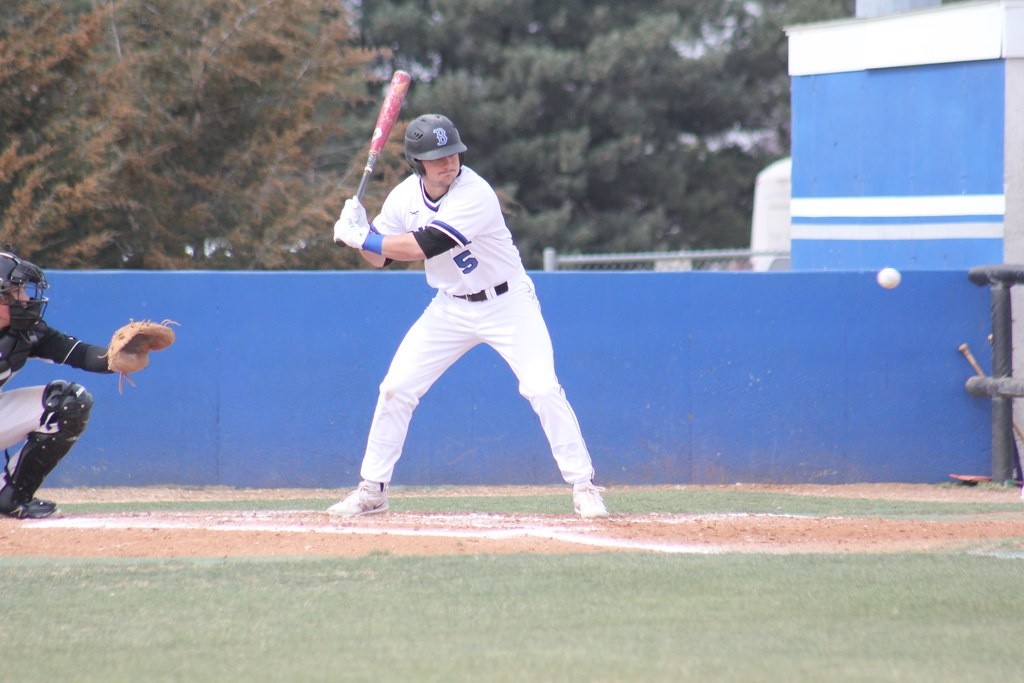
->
[106,321,174,374]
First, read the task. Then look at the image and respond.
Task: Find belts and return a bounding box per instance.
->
[454,282,507,302]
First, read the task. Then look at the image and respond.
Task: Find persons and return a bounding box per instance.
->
[0,251,174,519]
[327,113,609,522]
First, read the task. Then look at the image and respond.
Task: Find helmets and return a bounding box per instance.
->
[405,114,468,178]
[0,249,22,294]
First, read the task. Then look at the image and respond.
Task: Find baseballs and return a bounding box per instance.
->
[876,267,901,290]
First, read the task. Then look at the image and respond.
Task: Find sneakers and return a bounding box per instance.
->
[0,497,58,518]
[573,482,608,518]
[328,481,391,516]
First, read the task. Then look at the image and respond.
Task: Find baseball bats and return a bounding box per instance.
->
[958,342,1024,443]
[334,69,412,248]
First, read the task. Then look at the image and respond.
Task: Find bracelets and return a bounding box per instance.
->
[362,233,384,255]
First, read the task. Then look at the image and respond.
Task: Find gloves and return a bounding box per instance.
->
[333,196,370,250]
